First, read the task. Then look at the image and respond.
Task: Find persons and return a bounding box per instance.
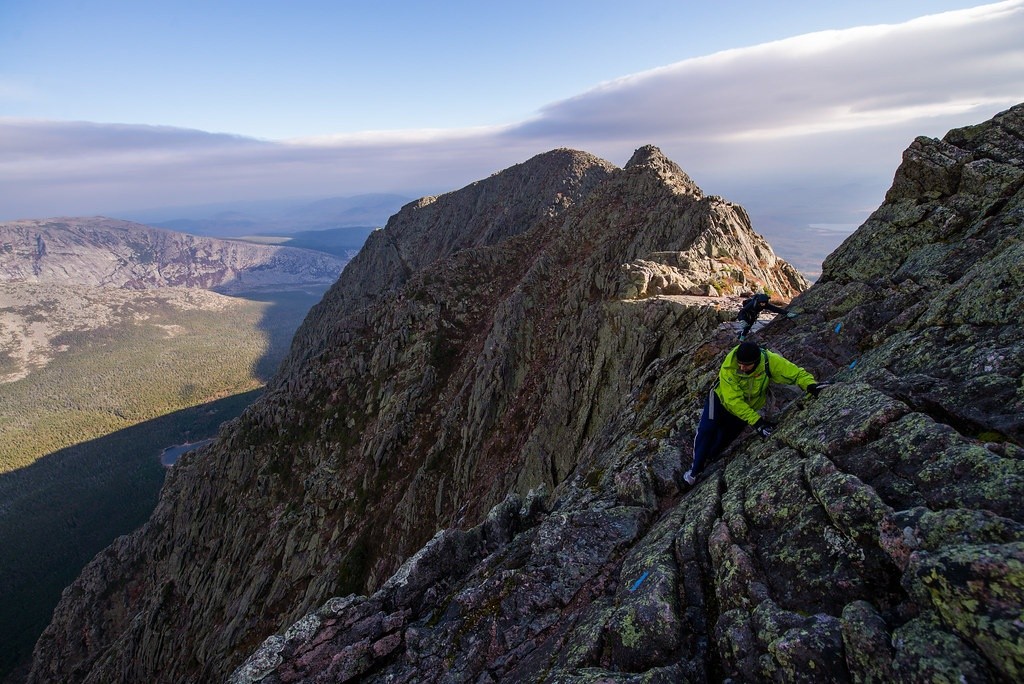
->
[736,294,798,341]
[684,341,833,485]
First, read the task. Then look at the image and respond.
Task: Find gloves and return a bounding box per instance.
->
[807,382,830,398]
[787,312,797,318]
[743,322,750,329]
[754,417,777,437]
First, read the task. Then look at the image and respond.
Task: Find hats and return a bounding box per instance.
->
[736,341,760,362]
[758,294,767,303]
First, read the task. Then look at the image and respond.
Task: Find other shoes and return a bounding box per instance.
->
[740,334,745,342]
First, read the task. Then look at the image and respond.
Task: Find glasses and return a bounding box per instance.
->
[738,361,756,366]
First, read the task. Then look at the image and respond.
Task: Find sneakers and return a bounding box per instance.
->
[683,470,698,485]
